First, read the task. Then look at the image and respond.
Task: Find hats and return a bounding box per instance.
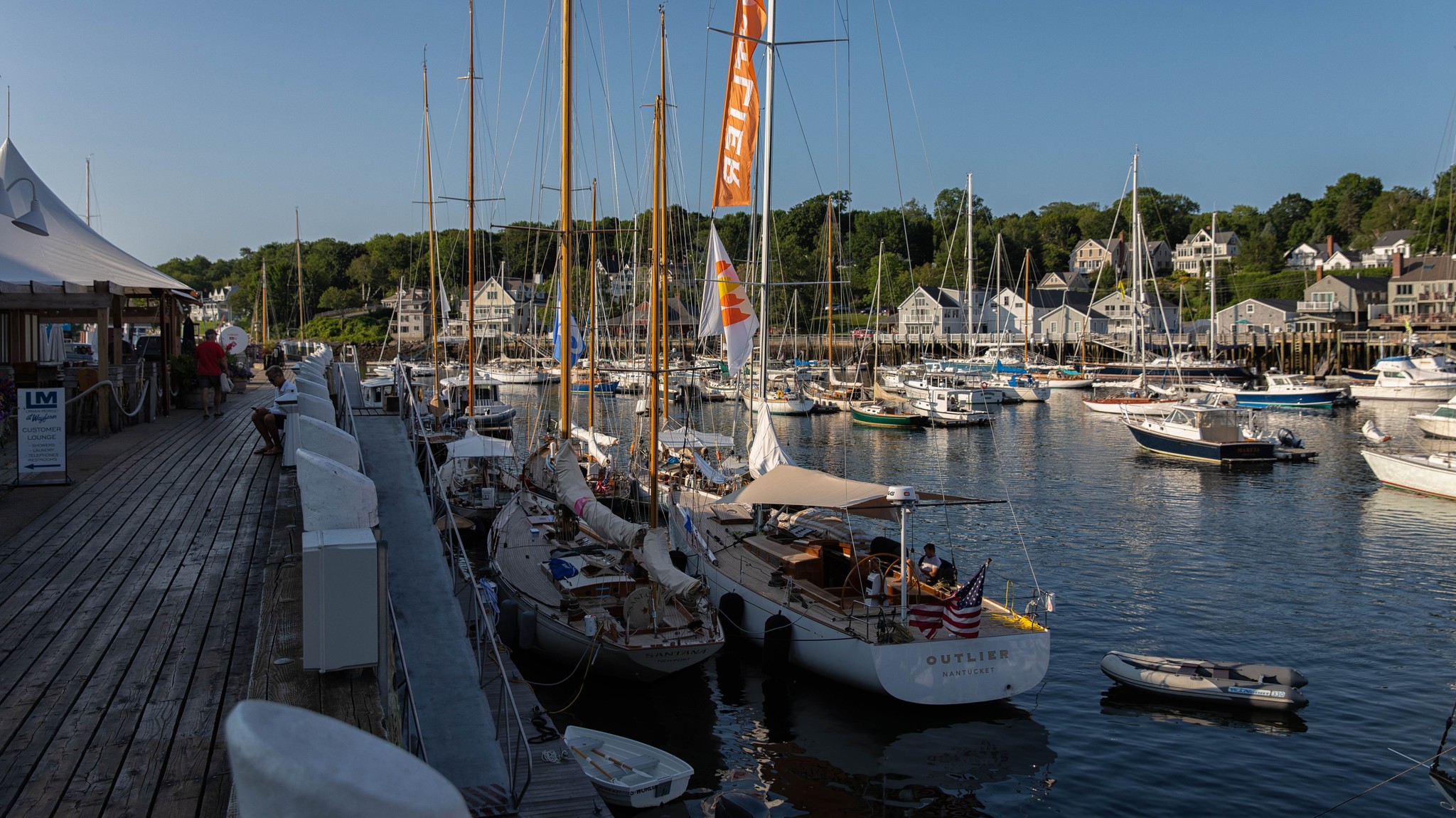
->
[205,329,220,336]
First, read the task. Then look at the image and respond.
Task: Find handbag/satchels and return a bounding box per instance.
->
[220,373,235,392]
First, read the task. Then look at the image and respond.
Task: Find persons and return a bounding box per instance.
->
[460,386,469,415]
[1134,391,1140,398]
[194,328,229,419]
[81,347,87,354]
[439,382,452,422]
[891,543,942,586]
[0,366,19,436]
[250,365,297,456]
[108,333,134,364]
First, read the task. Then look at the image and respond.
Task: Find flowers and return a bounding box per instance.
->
[221,342,256,380]
[169,348,199,389]
[0,372,18,444]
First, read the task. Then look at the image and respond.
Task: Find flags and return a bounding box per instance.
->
[596,470,610,493]
[909,563,988,640]
[697,218,760,378]
[418,389,423,404]
[552,280,586,369]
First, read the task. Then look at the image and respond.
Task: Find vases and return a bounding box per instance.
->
[230,377,247,394]
[183,386,216,410]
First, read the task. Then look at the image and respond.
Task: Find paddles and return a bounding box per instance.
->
[570,745,629,787]
[590,749,654,778]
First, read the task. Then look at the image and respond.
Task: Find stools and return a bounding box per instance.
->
[13,362,36,389]
[77,368,100,434]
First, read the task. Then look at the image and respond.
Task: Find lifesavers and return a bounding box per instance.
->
[685,474,696,489]
[981,382,987,389]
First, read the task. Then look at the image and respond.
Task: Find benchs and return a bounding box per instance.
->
[798,578,841,604]
[886,575,932,605]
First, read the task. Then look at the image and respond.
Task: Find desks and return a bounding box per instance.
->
[36,360,170,433]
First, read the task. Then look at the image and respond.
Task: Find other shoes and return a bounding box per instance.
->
[204,414,210,419]
[214,411,224,417]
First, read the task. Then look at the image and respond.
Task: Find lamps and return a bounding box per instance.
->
[0,176,48,236]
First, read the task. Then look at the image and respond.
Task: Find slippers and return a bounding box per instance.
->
[264,449,284,456]
[254,448,267,454]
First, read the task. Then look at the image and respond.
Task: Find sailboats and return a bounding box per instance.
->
[368,145,1456,437]
[1081,212,1191,417]
[252,0,1456,562]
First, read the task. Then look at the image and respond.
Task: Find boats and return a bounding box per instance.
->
[223,697,470,818]
[564,725,695,809]
[484,431,725,676]
[1358,305,1456,501]
[1118,379,1279,466]
[1239,407,1319,460]
[1099,647,1309,713]
[668,173,1052,704]
[1429,701,1456,812]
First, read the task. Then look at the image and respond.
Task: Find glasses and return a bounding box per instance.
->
[269,375,278,382]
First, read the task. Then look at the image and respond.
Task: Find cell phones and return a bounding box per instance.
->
[251,407,259,411]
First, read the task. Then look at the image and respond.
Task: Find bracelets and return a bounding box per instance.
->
[266,410,270,414]
